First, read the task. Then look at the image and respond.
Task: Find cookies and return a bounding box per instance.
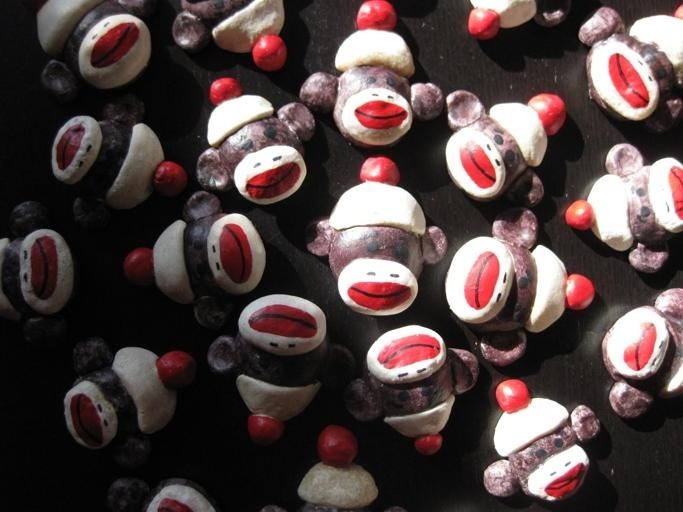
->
[0,0,683,512]
